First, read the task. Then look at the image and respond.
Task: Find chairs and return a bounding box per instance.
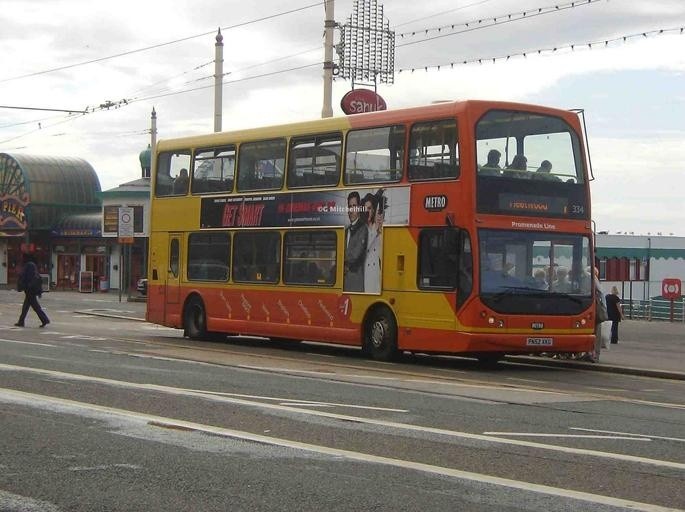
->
[174,162,461,193]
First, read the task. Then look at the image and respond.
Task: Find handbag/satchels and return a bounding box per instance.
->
[29,262,43,294]
[599,291,608,321]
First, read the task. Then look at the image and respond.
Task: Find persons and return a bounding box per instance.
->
[343,191,369,292]
[503,154,528,179]
[173,168,190,184]
[478,149,503,176]
[536,160,562,183]
[605,285,625,344]
[14,251,50,328]
[528,260,609,364]
[355,188,390,294]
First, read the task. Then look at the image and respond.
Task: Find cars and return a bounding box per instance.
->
[136,279,147,293]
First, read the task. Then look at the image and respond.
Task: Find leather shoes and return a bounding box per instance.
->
[15,323,24,327]
[40,321,49,327]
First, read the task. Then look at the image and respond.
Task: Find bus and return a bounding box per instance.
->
[145,100,596,363]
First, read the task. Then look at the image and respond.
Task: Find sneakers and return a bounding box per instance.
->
[532,352,598,364]
